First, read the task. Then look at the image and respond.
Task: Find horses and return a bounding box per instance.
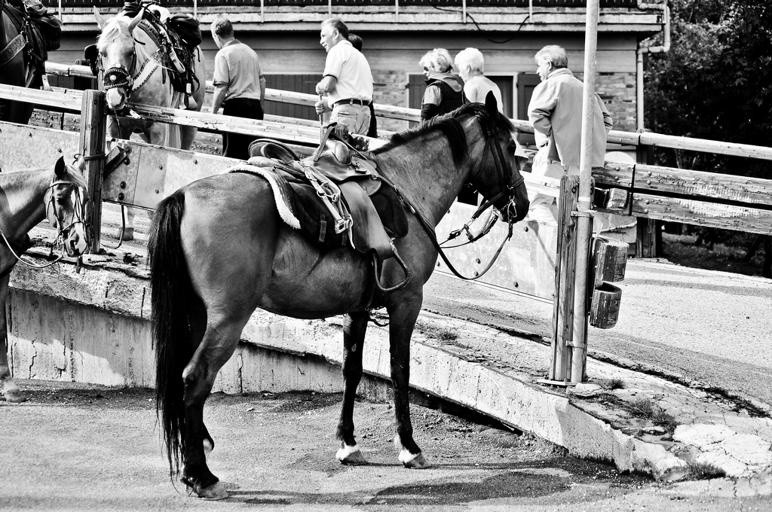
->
[0,1,44,126]
[92,4,207,242]
[0,155,93,406]
[146,90,530,502]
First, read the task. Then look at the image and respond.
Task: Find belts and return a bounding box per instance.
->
[332,99,370,110]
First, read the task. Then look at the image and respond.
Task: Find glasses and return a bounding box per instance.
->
[422,65,435,72]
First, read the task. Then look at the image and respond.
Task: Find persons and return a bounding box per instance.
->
[527,44,614,182]
[454,46,505,116]
[418,46,479,206]
[314,16,373,136]
[347,32,378,138]
[210,15,267,160]
